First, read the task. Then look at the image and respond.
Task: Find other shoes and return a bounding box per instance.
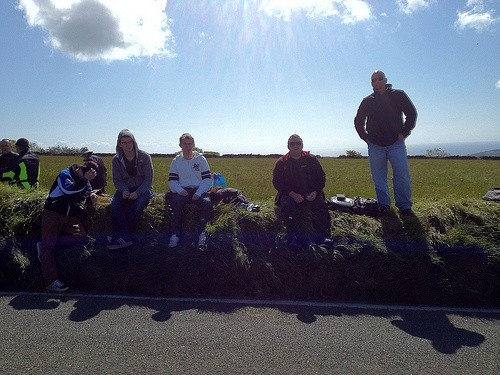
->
[379,202,412,214]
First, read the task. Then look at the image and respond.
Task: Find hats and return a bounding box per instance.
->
[79,146,93,157]
[15,137,30,147]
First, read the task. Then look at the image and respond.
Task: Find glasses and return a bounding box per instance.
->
[372,76,384,82]
[289,141,302,146]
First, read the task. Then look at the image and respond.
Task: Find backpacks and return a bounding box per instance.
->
[352,194,378,216]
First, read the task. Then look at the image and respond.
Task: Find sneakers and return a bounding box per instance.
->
[37,241,42,263]
[168,235,180,247]
[46,280,69,292]
[197,233,206,246]
[106,233,133,249]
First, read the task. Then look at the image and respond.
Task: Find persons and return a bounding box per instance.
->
[79,147,107,195]
[353,69,417,216]
[272,134,334,247]
[7,137,42,191]
[0,138,20,184]
[105,128,154,249]
[37,160,99,291]
[167,132,212,248]
